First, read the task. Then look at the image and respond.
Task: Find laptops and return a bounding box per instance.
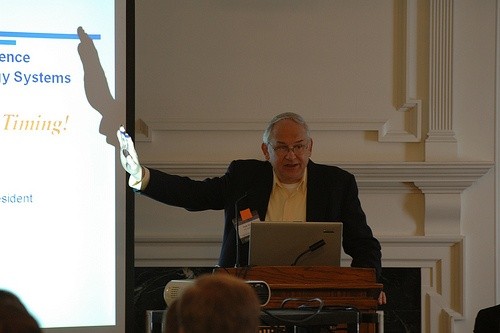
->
[248,221,343,267]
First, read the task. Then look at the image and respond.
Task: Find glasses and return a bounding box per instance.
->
[268,140,309,152]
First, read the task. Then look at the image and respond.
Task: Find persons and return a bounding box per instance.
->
[160,274,262,333]
[116,110,387,304]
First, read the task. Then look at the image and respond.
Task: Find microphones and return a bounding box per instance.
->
[234,189,255,267]
